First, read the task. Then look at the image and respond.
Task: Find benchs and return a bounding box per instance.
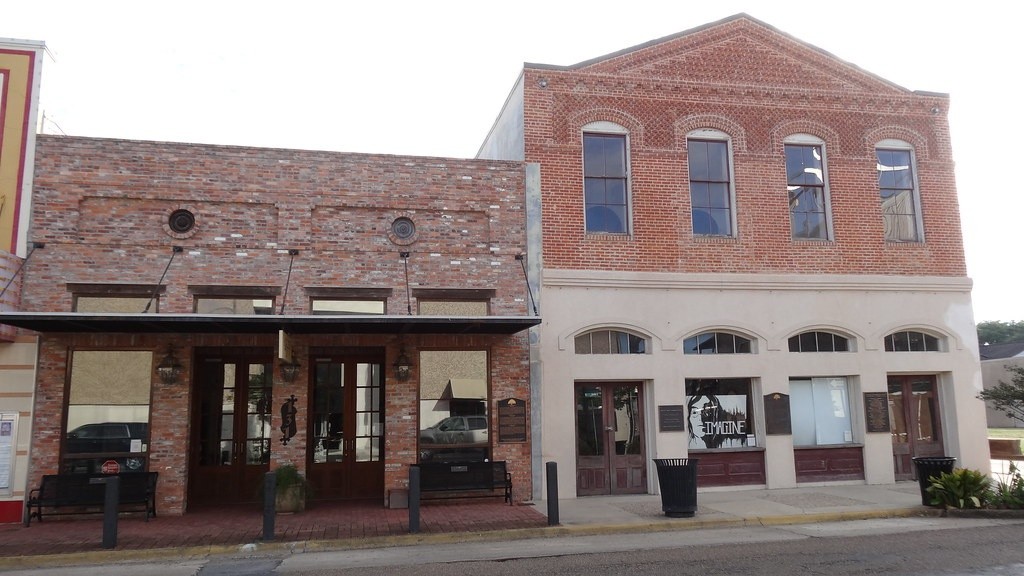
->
[409,461,513,506]
[24,471,159,527]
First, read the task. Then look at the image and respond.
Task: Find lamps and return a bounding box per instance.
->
[277,350,302,384]
[393,348,414,382]
[155,343,186,386]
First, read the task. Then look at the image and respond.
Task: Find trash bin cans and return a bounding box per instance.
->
[651,457,703,519]
[911,454,959,507]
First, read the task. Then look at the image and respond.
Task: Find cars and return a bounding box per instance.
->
[420,413,489,446]
[66,420,147,454]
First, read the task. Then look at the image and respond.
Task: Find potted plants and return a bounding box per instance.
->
[274,464,314,513]
